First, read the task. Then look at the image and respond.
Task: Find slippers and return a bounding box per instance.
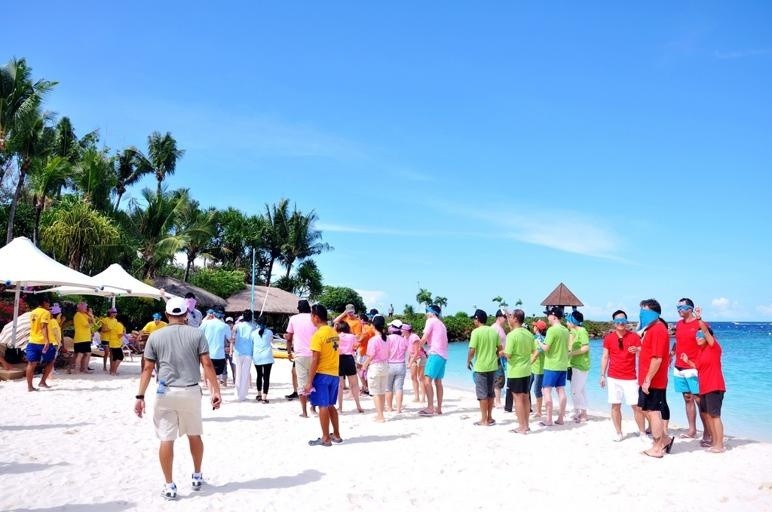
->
[329,433,342,443]
[418,410,434,416]
[612,430,725,458]
[308,438,332,446]
[473,408,590,433]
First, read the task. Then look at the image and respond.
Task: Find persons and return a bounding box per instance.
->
[133,295,222,501]
[26,294,127,393]
[130,291,274,403]
[598,296,727,458]
[282,299,450,447]
[467,306,591,436]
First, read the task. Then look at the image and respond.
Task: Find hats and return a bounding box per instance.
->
[565,313,583,326]
[366,312,375,318]
[470,310,487,323]
[166,297,188,316]
[345,304,355,313]
[532,321,546,330]
[226,317,233,322]
[387,319,403,328]
[543,307,563,317]
[496,308,508,319]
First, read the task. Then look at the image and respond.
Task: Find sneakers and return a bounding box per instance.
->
[161,483,177,500]
[256,396,262,401]
[191,473,202,491]
[285,392,299,400]
[261,400,269,404]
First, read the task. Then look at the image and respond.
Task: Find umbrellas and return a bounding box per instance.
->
[47,262,181,308]
[0,235,105,348]
